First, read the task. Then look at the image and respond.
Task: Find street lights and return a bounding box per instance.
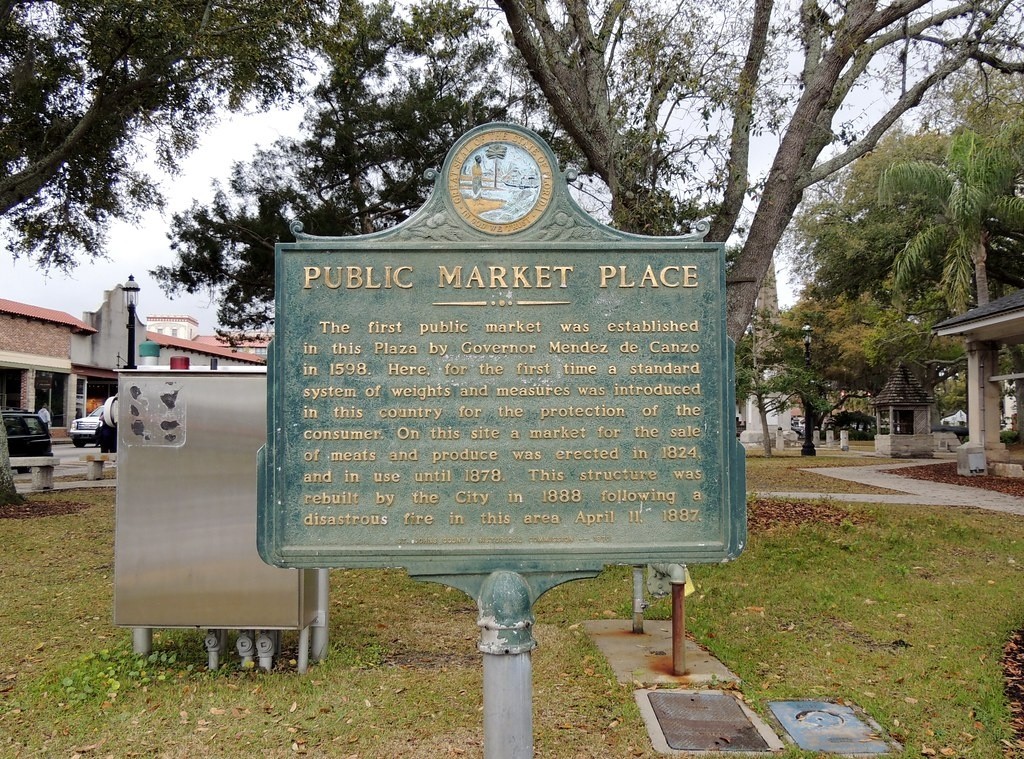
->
[120,274,142,369]
[801,322,817,457]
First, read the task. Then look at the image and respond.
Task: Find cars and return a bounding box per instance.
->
[790,416,895,440]
[1000,417,1012,430]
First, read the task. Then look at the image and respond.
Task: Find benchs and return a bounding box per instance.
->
[9,457,60,490]
[79,453,117,480]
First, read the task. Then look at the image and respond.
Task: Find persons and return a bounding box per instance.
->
[97,412,118,453]
[37,401,52,437]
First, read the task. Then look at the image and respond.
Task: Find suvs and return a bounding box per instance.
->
[0,405,54,474]
[69,405,105,448]
